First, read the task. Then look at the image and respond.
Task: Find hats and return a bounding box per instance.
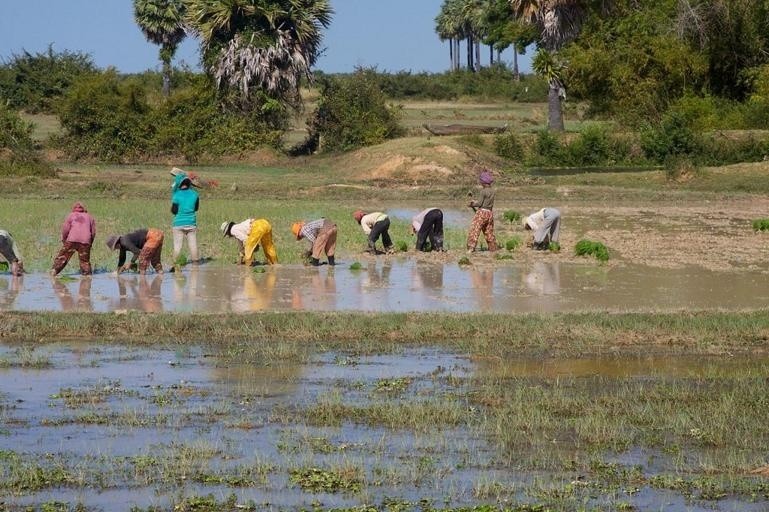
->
[221,221,232,237]
[105,234,120,252]
[291,221,306,241]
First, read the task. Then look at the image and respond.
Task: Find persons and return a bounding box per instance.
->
[359,260,395,294]
[108,275,167,317]
[0,230,21,275]
[49,200,97,276]
[291,218,337,265]
[219,218,280,268]
[465,171,499,251]
[223,268,277,312]
[354,209,394,256]
[171,172,200,264]
[413,256,444,302]
[468,255,497,307]
[176,271,202,311]
[309,268,339,309]
[522,206,562,250]
[5,277,19,307]
[527,256,561,294]
[56,277,98,311]
[411,207,445,252]
[106,227,165,274]
